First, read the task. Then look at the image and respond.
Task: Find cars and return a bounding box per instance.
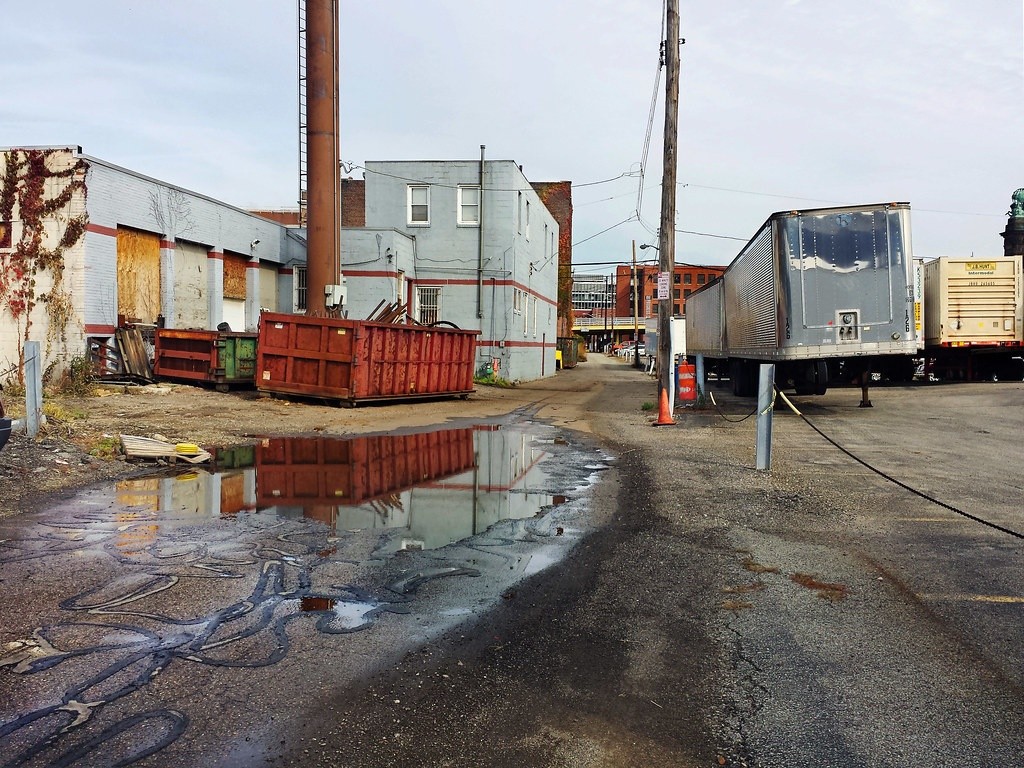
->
[620,345,645,356]
[610,341,635,354]
[599,339,610,353]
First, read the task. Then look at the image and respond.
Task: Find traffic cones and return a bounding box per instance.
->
[653,388,676,426]
